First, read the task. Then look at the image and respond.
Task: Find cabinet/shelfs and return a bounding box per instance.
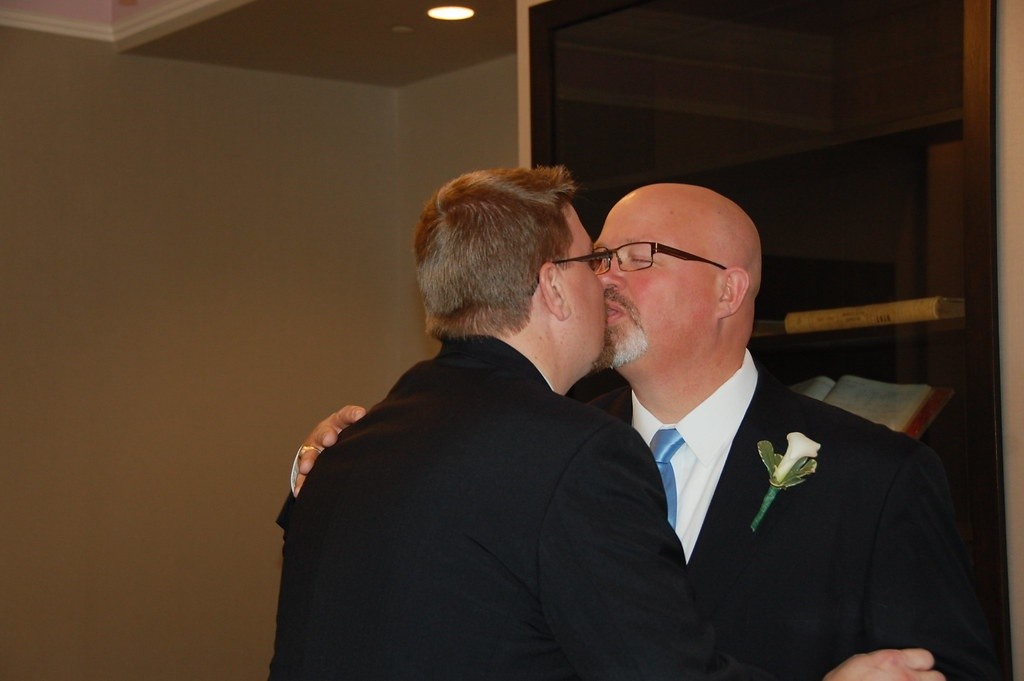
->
[514,0,1005,600]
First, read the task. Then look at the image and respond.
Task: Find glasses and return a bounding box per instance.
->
[604,241,728,276]
[536,246,611,285]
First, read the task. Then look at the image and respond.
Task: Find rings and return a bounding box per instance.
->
[297,444,321,453]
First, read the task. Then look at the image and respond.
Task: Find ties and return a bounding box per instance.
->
[649,428,686,534]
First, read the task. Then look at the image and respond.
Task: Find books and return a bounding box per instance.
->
[783,295,962,335]
[788,375,956,443]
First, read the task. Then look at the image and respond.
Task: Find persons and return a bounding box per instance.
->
[288,181,1001,681]
[268,166,948,681]
